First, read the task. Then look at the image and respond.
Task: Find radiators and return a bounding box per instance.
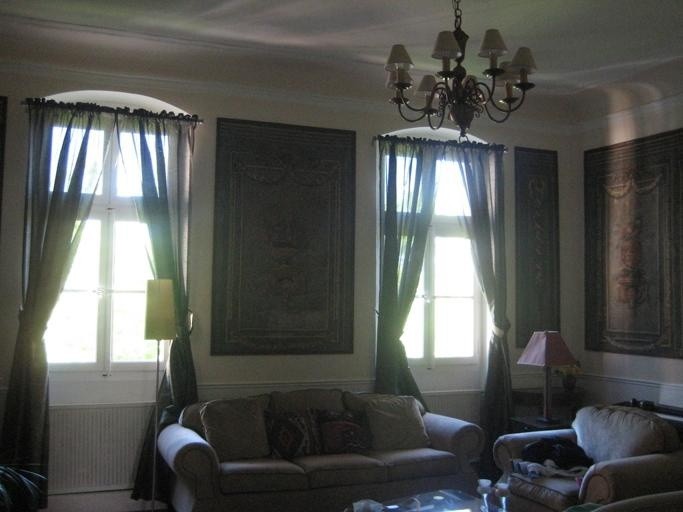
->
[47,402,155,497]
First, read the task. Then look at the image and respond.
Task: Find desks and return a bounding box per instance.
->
[508,413,574,433]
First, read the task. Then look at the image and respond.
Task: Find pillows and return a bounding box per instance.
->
[263,406,323,458]
[344,391,432,451]
[272,387,348,413]
[199,397,271,462]
[308,407,369,456]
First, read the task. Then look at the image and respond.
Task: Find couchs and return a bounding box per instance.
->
[156,392,486,511]
[491,401,683,511]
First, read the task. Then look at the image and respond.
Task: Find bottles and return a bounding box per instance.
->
[476,478,492,512]
[493,483,510,512]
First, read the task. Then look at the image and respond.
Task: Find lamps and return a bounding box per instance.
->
[143,278,177,512]
[383,1,536,147]
[516,329,577,425]
